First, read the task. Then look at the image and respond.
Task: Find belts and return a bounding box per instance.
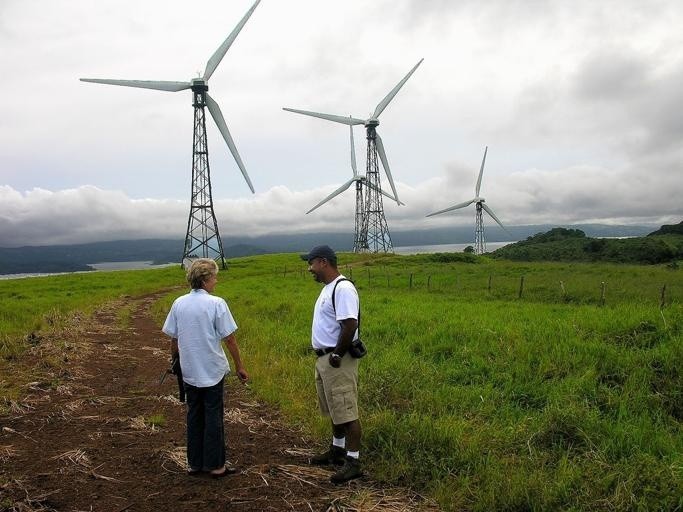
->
[315,347,336,357]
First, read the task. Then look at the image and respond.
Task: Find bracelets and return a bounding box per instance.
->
[329,352,343,360]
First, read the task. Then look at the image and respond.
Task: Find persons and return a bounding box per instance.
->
[301,243,365,483]
[160,258,249,478]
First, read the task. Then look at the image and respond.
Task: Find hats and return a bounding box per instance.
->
[299,245,337,262]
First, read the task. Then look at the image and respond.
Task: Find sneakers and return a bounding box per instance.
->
[330,456,362,483]
[310,443,347,464]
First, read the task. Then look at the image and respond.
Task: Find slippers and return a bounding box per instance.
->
[188,465,203,475]
[209,465,237,478]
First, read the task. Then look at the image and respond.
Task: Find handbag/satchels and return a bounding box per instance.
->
[350,338,367,358]
